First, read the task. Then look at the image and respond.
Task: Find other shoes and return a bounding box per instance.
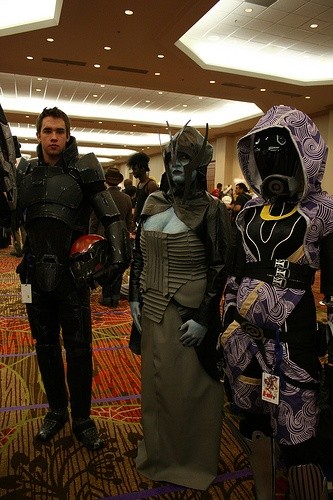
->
[71,415,104,450]
[10,250,17,256]
[36,410,69,443]
[98,297,120,307]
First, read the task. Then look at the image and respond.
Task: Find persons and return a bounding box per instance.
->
[208,183,260,214]
[217,104,333,500]
[7,220,26,255]
[128,119,234,491]
[2,105,128,446]
[100,152,170,306]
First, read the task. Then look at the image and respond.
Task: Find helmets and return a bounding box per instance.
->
[68,234,112,289]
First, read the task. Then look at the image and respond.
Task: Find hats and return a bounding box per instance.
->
[222,184,232,194]
[105,167,124,182]
